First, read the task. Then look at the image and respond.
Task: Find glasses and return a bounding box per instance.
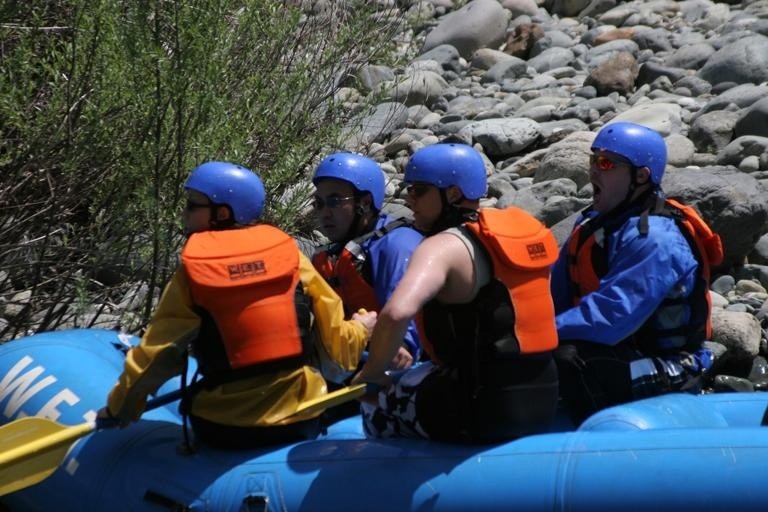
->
[186,198,209,211]
[407,182,430,197]
[310,193,354,210]
[588,154,627,172]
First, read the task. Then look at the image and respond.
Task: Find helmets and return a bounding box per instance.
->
[404,141,488,202]
[182,160,266,225]
[311,151,385,212]
[590,121,669,190]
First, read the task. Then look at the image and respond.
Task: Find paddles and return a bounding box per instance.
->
[0,379,204,496]
[269,359,428,426]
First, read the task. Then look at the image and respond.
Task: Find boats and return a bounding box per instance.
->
[0,327,768,511]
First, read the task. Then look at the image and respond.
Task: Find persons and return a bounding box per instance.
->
[305,153,423,423]
[352,142,559,443]
[91,160,378,451]
[550,121,713,417]
